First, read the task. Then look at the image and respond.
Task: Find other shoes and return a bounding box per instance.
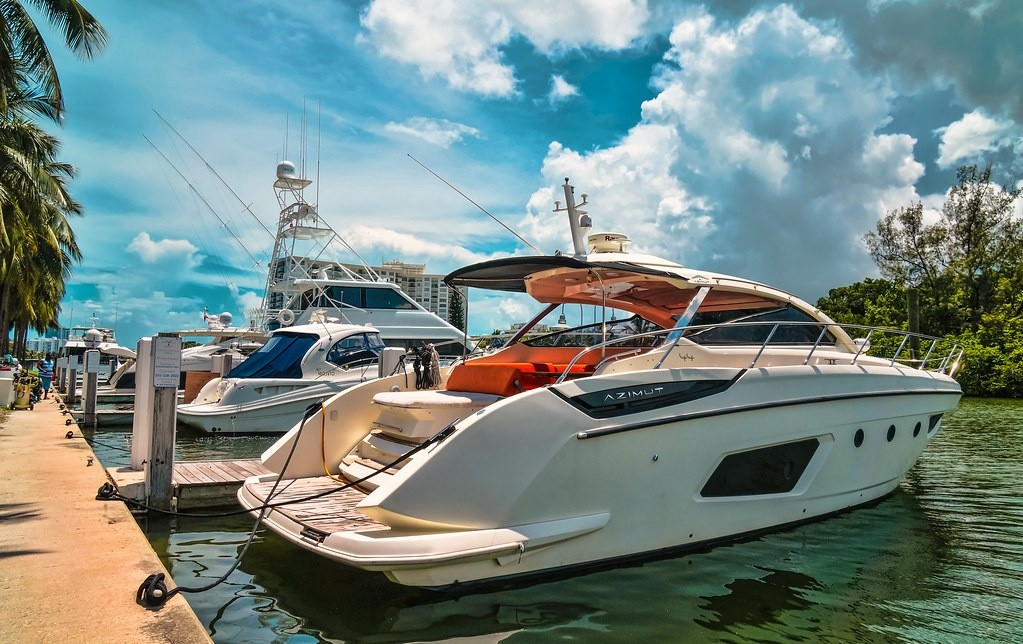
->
[44,397,50,399]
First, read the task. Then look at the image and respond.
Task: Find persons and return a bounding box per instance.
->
[38,352,54,400]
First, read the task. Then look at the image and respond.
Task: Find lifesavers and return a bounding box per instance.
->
[216,380,231,399]
[278,308,295,326]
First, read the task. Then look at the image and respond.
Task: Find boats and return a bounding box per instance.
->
[239,486,947,644]
[65,93,503,437]
[236,177,961,603]
